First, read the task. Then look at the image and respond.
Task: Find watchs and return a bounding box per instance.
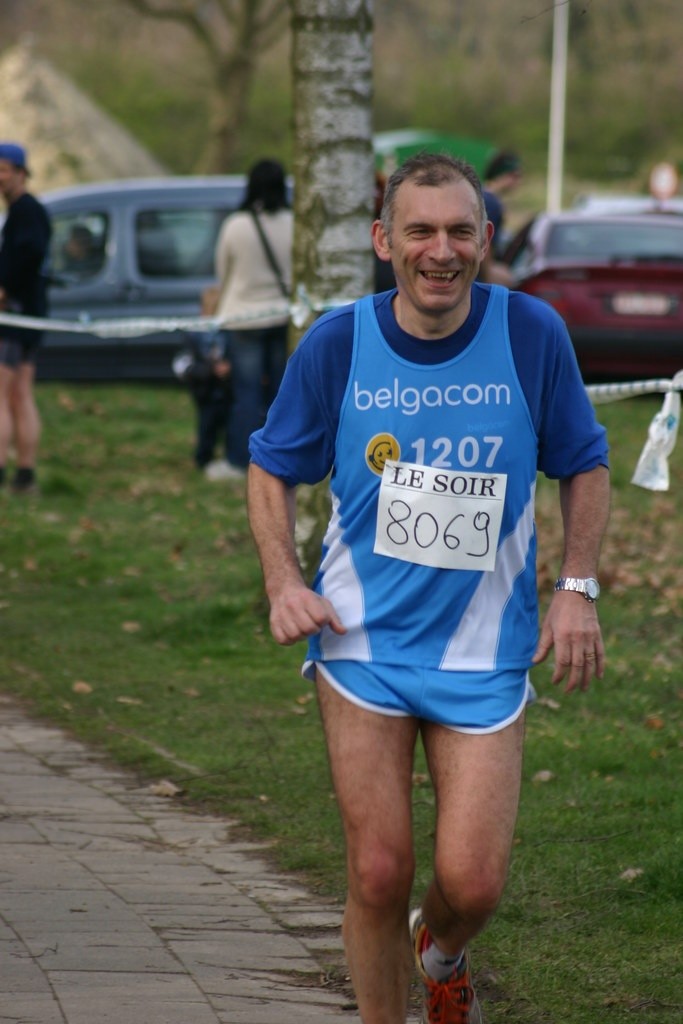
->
[554,576,602,603]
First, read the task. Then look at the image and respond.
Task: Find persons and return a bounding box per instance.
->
[66,223,104,277]
[202,156,295,481]
[480,150,524,285]
[0,143,55,492]
[244,147,610,1023]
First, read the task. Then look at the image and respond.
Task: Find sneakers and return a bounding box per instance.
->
[409,909,482,1024]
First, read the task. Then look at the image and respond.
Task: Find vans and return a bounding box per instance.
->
[25,172,293,382]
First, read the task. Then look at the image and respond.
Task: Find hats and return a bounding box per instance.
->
[0,144,24,167]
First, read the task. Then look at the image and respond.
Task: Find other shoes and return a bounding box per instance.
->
[206,460,248,483]
[12,480,37,498]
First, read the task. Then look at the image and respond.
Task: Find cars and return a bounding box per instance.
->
[502,211,683,376]
